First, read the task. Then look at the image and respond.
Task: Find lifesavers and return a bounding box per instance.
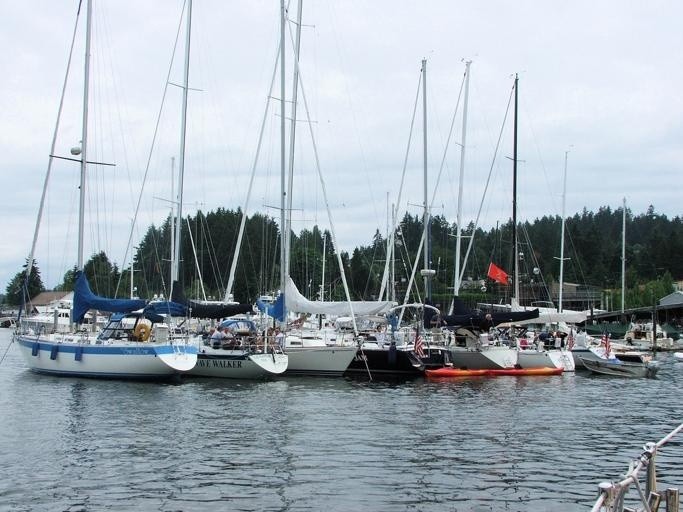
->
[135,324,150,341]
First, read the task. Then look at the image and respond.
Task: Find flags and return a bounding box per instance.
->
[568,327,576,350]
[601,327,611,359]
[488,262,506,284]
[415,329,424,357]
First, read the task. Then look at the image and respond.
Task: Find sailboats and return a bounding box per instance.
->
[447,61,520,371]
[517,72,574,372]
[345,57,449,381]
[557,150,615,368]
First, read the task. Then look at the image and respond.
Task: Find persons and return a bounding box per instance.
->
[271,327,283,352]
[479,314,491,332]
[207,328,215,345]
[423,297,433,329]
[408,327,417,345]
[555,329,567,348]
[268,327,272,335]
[430,310,447,344]
[210,327,222,348]
[539,326,554,350]
[373,320,388,347]
[220,327,239,349]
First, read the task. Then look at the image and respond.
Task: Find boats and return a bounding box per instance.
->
[23,309,71,333]
[285,1,454,377]
[624,323,683,352]
[571,312,624,369]
[448,73,576,373]
[425,365,566,377]
[397,59,519,369]
